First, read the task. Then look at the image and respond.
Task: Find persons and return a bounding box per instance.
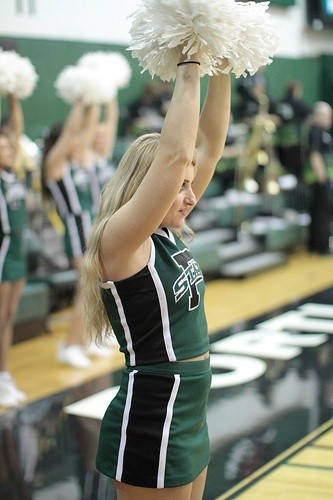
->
[79,2,283,500]
[124,81,333,254]
[1,50,131,408]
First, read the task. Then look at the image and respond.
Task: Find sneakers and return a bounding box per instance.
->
[102,330,118,345]
[57,343,92,368]
[82,341,113,358]
[0,382,18,406]
[4,382,26,401]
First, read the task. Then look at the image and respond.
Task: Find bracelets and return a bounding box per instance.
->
[175,60,201,67]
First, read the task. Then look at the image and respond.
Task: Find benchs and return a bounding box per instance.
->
[0,116,333,347]
